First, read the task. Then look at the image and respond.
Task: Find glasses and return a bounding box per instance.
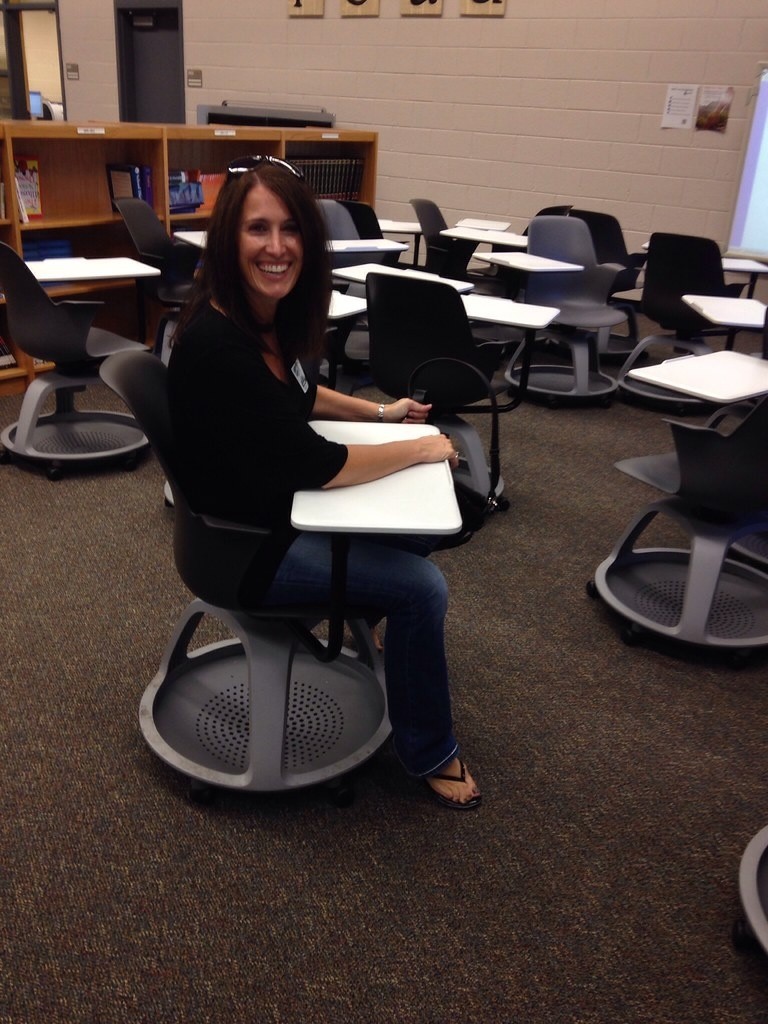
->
[225,153,304,179]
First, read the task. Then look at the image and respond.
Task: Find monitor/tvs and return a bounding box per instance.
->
[28,92,43,121]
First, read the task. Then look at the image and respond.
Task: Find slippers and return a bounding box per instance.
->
[420,756,485,810]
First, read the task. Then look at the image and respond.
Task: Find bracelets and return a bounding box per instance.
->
[378,403,385,421]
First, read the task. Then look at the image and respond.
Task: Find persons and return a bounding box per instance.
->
[168,154,482,809]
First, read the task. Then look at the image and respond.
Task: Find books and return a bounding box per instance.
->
[0,182,5,220]
[169,168,201,213]
[0,336,47,370]
[15,177,30,223]
[107,162,152,213]
[287,155,365,201]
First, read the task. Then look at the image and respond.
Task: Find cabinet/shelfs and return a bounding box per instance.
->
[0,119,380,383]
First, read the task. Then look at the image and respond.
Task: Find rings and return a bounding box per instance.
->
[456,452,460,458]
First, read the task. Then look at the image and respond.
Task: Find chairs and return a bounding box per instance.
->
[0,196,768,965]
